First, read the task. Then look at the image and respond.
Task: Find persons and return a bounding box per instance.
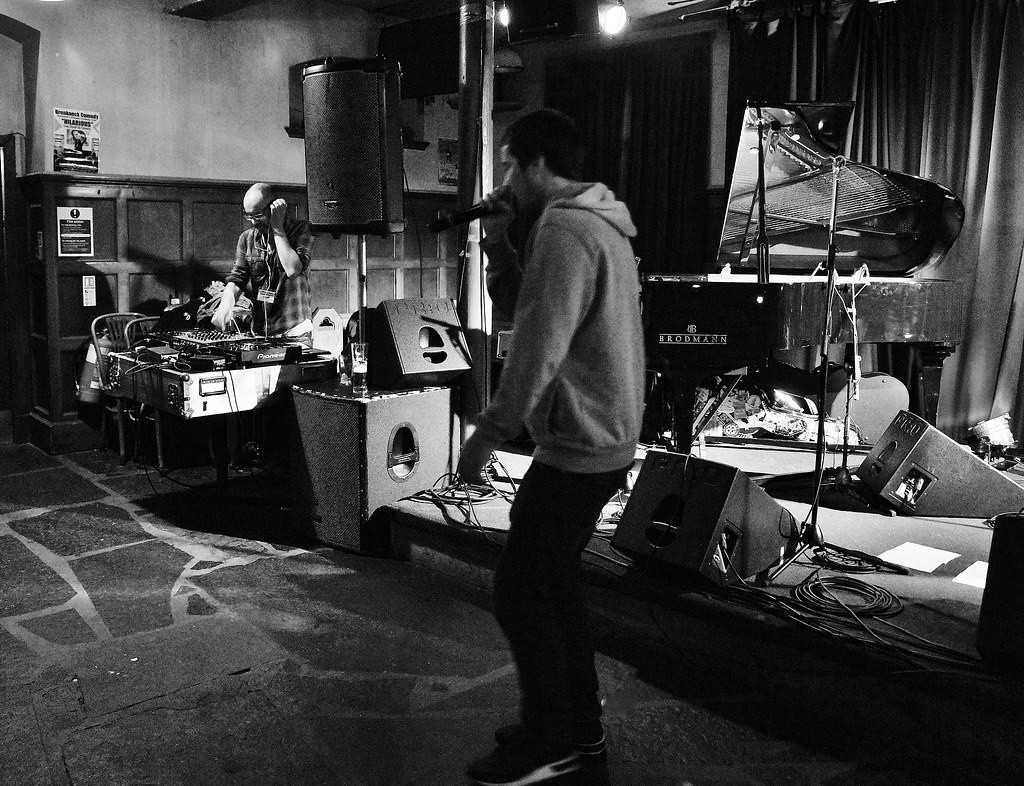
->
[458,109,648,786]
[211,181,316,350]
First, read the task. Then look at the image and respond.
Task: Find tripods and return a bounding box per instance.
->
[785,157,903,584]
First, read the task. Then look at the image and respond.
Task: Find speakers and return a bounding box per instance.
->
[280,381,453,552]
[857,411,1022,517]
[377,298,474,384]
[615,449,799,585]
[298,57,406,236]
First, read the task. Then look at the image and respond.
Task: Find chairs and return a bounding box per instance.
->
[124,316,218,471]
[91,312,155,465]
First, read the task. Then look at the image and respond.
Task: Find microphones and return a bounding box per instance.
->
[428,186,510,237]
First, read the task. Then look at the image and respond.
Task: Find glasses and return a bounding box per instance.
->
[242,198,276,221]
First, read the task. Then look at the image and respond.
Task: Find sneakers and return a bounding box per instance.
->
[464,721,604,785]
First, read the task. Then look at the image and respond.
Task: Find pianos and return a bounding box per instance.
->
[638,96,971,458]
